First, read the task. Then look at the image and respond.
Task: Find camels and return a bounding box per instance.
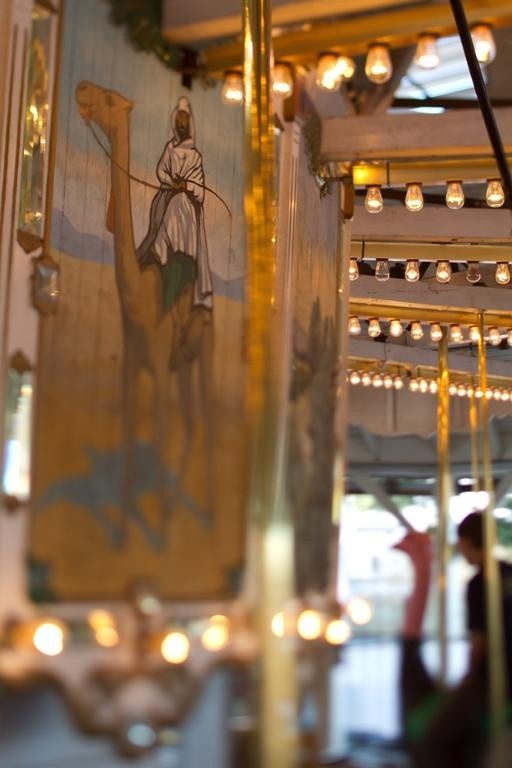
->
[75,80,220,554]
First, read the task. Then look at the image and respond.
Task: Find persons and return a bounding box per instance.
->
[423,511,511,768]
[135,96,214,311]
